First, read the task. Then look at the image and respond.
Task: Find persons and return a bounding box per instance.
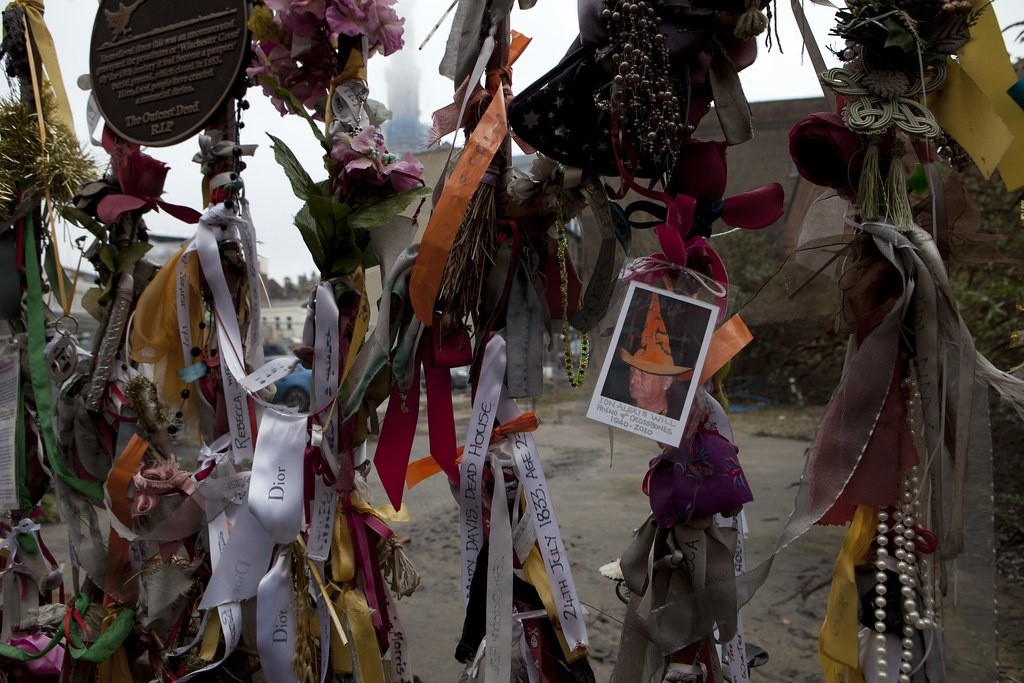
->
[629,365,678,414]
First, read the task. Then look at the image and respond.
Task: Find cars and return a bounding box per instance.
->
[263,337,314,413]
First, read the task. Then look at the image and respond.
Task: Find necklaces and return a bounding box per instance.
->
[876,378,934,683]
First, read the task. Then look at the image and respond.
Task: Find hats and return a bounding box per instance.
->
[619,292,693,376]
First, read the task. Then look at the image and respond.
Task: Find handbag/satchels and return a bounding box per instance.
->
[505,42,688,179]
